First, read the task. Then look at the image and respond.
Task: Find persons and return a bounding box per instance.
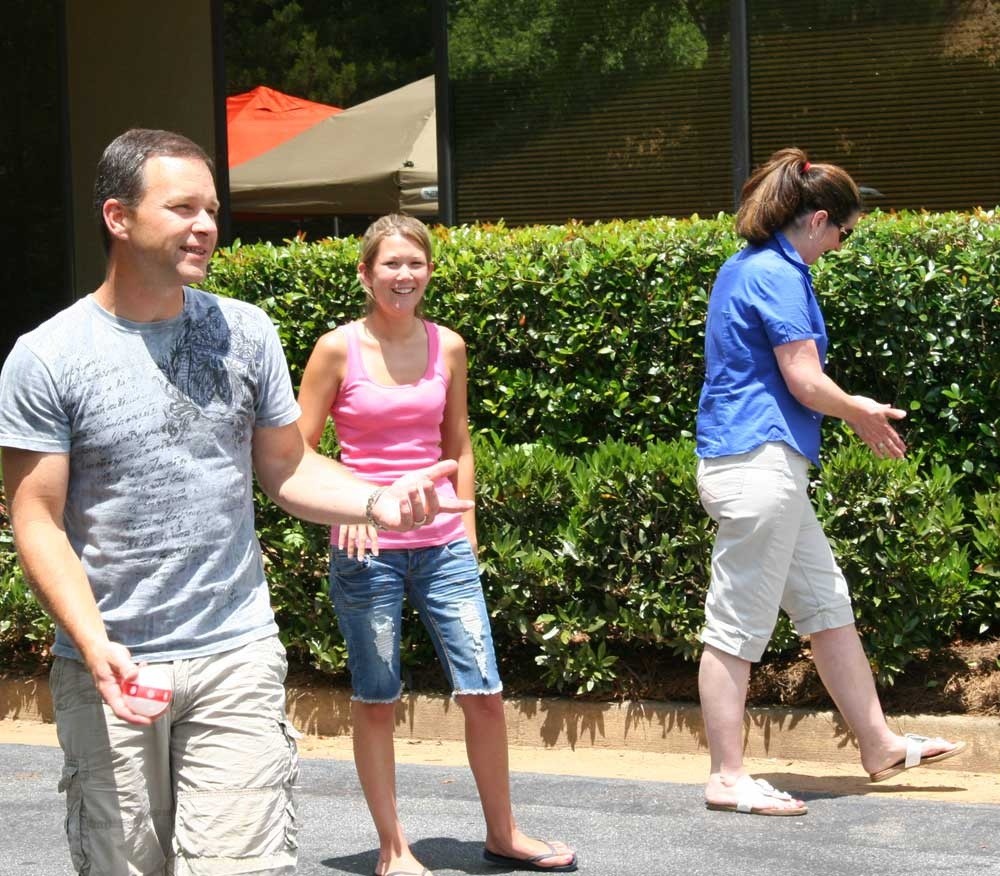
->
[696,148,965,816]
[0,129,477,876]
[298,213,578,876]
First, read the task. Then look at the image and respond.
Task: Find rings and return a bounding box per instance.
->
[413,515,427,526]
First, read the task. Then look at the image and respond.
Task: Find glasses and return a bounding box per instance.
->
[819,209,854,243]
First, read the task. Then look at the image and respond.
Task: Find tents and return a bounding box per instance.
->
[222,73,436,220]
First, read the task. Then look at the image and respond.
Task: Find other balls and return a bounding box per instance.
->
[122,665,173,718]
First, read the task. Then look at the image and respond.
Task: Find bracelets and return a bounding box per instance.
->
[367,484,385,534]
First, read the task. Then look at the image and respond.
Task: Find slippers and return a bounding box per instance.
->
[373,862,430,875]
[871,733,966,783]
[706,769,809,817]
[483,833,579,872]
